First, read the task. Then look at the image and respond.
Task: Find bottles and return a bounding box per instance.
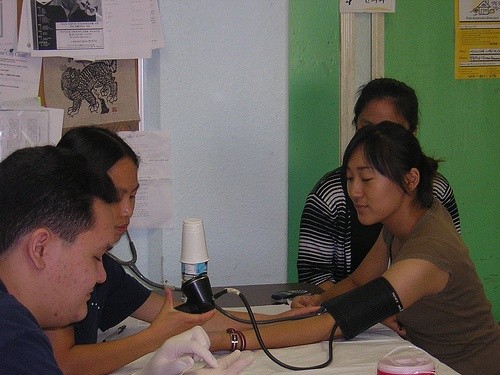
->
[181,262,208,286]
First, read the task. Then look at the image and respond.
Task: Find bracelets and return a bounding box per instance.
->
[227,327,238,352]
[237,330,247,351]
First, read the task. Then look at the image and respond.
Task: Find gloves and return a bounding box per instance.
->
[184,350,256,375]
[132,326,218,375]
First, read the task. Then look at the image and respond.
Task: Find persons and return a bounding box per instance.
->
[53,125,324,344]
[294,77,461,342]
[204,120,499,375]
[1,144,217,375]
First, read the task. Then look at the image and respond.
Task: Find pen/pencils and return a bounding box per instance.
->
[103,325,126,342]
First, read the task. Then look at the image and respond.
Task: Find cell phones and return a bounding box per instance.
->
[271,290,308,303]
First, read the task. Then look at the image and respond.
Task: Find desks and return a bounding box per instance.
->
[95,282,462,375]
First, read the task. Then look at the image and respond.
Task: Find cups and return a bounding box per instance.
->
[180,218,209,264]
